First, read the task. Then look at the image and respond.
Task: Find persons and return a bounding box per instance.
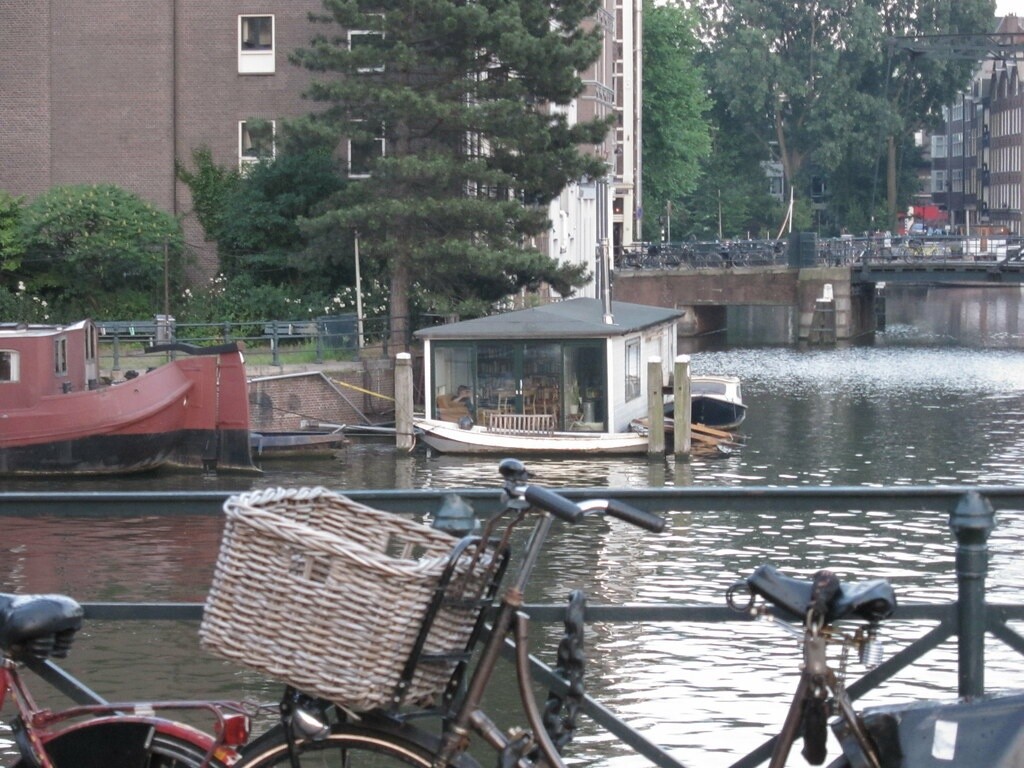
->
[450,385,478,424]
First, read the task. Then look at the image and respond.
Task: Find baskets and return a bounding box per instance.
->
[197,483,505,715]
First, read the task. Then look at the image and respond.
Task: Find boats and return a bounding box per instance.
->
[248,427,352,455]
[413,295,751,460]
[1,316,265,478]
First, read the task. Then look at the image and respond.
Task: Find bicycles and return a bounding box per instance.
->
[613,232,948,265]
[0,589,254,768]
[193,461,898,768]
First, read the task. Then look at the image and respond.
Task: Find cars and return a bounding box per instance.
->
[910,224,946,238]
